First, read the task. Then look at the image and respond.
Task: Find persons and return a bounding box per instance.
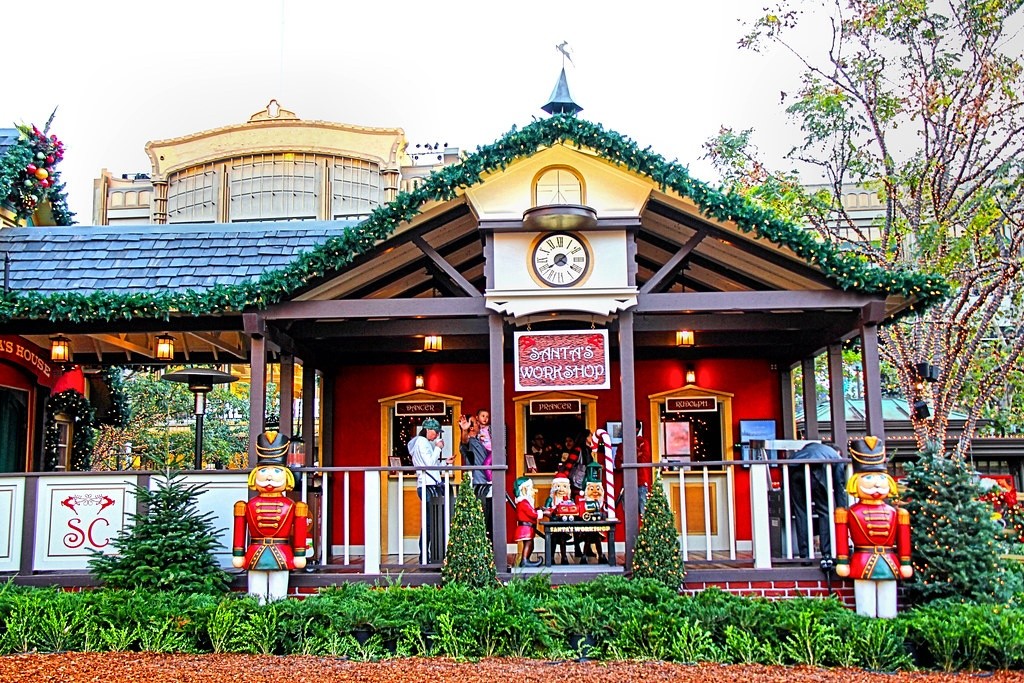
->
[230,458,313,606]
[475,407,491,499]
[512,476,553,568]
[562,428,596,493]
[526,431,549,468]
[785,440,850,566]
[558,433,578,469]
[582,478,605,507]
[833,467,914,620]
[407,417,457,567]
[544,476,574,512]
[455,412,493,546]
[612,420,653,549]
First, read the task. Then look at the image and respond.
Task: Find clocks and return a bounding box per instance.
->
[530,230,589,288]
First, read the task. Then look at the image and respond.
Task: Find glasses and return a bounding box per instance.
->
[534,438,543,440]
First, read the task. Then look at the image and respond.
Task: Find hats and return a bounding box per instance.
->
[422,418,445,433]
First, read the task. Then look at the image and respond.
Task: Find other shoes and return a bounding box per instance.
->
[800,556,811,565]
[575,548,583,557]
[583,545,597,557]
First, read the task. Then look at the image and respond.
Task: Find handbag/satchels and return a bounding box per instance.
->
[558,448,586,490]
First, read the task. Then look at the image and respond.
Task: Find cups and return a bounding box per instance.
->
[435,438,442,444]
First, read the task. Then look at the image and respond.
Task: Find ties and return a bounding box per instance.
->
[535,452,540,460]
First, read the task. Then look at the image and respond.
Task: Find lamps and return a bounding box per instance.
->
[49,334,71,363]
[686,370,695,383]
[154,333,177,360]
[423,335,442,352]
[414,373,424,389]
[675,331,693,347]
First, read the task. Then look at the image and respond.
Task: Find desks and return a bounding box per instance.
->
[539,518,619,567]
